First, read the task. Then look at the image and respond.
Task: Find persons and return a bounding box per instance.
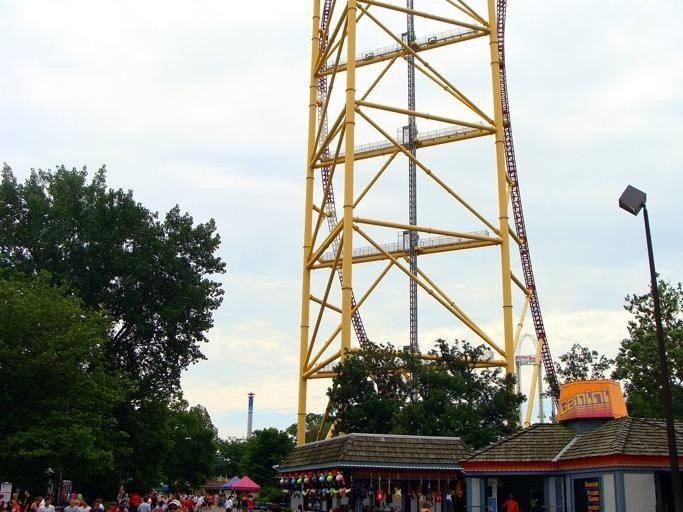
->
[0,481,257,511]
[502,493,519,512]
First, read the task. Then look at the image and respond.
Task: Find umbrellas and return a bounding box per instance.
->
[222,476,241,494]
[230,475,260,495]
